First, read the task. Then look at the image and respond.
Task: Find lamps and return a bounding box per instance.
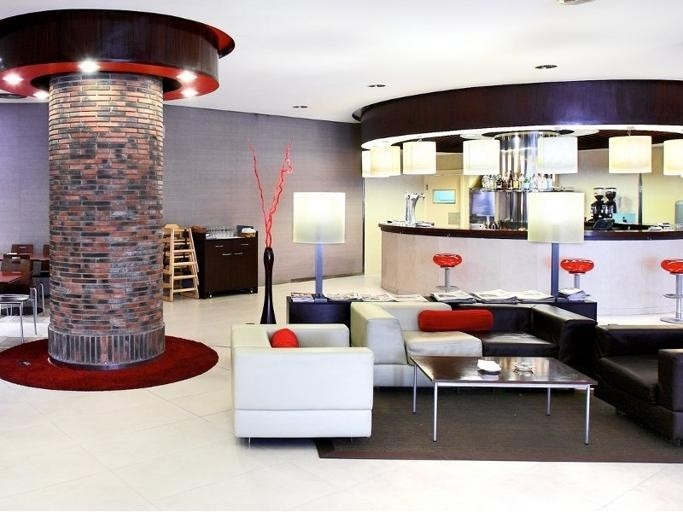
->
[527,191,585,296]
[663,138,682,176]
[362,146,401,178]
[463,140,499,176]
[538,136,578,175]
[402,141,436,175]
[609,135,652,173]
[293,191,346,296]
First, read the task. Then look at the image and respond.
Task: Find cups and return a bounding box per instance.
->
[471,224,485,231]
[205,224,235,238]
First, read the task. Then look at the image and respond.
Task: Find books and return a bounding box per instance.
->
[291,289,589,303]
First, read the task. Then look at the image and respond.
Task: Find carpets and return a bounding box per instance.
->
[313,387,683,460]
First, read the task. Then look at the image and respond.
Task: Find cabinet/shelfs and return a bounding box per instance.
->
[183,230,258,298]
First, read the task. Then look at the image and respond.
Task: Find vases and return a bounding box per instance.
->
[261,247,276,324]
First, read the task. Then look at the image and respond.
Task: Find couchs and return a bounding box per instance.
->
[350,300,598,387]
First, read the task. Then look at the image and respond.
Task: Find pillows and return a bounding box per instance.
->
[269,329,299,348]
[418,310,493,332]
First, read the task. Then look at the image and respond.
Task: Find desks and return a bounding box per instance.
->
[286,293,598,324]
[0,270,24,285]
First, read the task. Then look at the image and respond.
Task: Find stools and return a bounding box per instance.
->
[660,258,683,323]
[162,223,199,301]
[560,258,594,289]
[433,252,461,291]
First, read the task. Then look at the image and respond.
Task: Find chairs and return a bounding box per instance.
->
[229,323,374,438]
[0,243,50,344]
[594,324,683,446]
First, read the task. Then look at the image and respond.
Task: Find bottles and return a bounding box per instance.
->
[489,169,554,191]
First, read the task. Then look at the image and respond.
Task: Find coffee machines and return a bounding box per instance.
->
[590,187,605,213]
[601,187,616,213]
